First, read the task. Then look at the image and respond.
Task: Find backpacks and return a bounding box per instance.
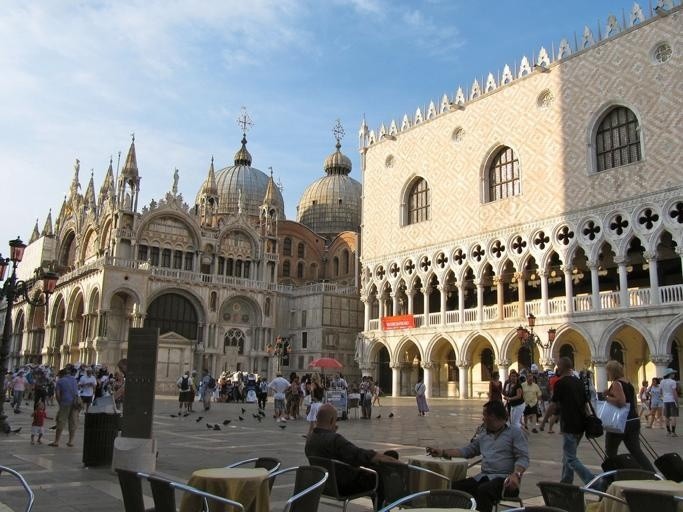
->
[181,376,188,390]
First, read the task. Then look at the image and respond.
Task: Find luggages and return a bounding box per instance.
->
[587,438,641,482]
[638,432,683,482]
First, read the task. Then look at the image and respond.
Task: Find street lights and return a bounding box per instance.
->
[266,334,290,372]
[0,236,59,432]
[516,313,555,367]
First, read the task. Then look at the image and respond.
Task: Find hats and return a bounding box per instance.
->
[663,368,677,377]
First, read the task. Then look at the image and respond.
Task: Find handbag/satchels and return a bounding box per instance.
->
[74,397,84,411]
[596,381,630,433]
[585,414,603,438]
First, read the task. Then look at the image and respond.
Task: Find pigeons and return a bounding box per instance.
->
[49,425,56,430]
[10,427,22,433]
[169,414,178,418]
[239,408,266,423]
[206,419,231,430]
[376,414,381,419]
[279,425,286,429]
[196,416,204,422]
[25,401,29,406]
[388,412,394,417]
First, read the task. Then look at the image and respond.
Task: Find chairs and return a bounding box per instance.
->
[584,467,664,487]
[502,505,567,511]
[227,456,282,496]
[537,481,628,512]
[0,465,34,512]
[263,466,330,511]
[115,467,246,511]
[468,458,525,511]
[373,454,451,511]
[379,487,476,511]
[307,455,379,512]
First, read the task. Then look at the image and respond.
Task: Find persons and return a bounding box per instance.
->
[306,389,324,432]
[176,369,211,416]
[639,368,680,437]
[268,372,380,421]
[415,379,429,416]
[602,361,656,472]
[248,374,268,410]
[541,358,597,484]
[304,403,405,510]
[487,365,593,433]
[5,359,125,446]
[427,401,530,510]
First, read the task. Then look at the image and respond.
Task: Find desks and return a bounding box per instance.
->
[304,387,362,422]
[597,480,683,511]
[180,468,271,511]
[400,455,469,506]
